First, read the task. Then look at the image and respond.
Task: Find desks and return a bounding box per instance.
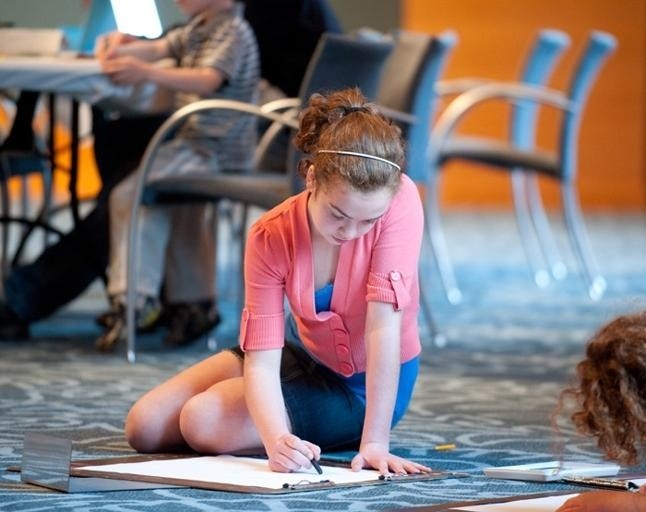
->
[0,57,178,312]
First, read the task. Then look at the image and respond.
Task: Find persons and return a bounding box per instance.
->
[123,85,435,479]
[557,309,645,511]
[0,0,350,344]
[93,1,262,352]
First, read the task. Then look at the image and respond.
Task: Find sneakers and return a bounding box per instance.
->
[94,300,221,354]
[0,301,30,341]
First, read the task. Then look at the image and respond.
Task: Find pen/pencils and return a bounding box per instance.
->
[311,459,322,474]
[435,444,456,451]
